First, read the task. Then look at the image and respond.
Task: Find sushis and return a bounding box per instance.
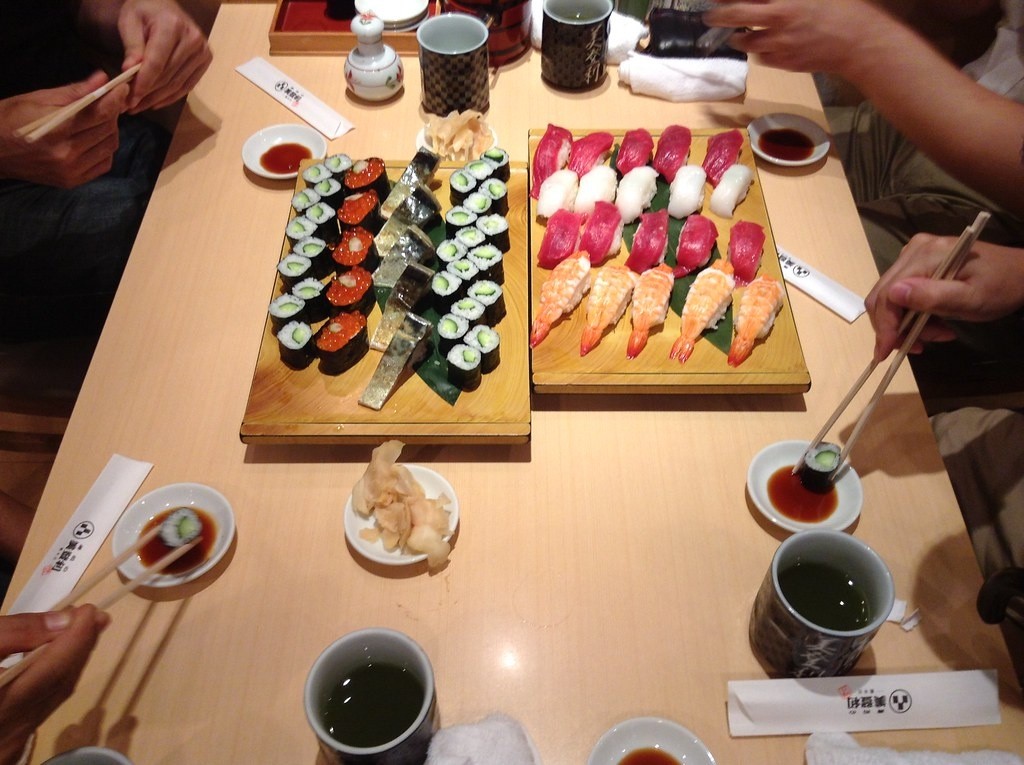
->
[159,507,202,550]
[531,124,786,365]
[801,442,842,492]
[268,153,390,373]
[358,146,511,410]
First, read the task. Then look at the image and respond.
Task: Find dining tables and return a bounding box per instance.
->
[0,0,1024,765]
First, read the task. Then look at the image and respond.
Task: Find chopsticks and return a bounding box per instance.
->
[12,61,143,143]
[789,209,992,481]
[0,517,205,686]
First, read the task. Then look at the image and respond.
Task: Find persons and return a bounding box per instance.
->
[865,232,1024,687]
[1,490,112,765]
[702,0,1024,362]
[0,0,212,387]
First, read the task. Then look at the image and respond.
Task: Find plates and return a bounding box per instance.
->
[746,439,863,533]
[353,0,429,33]
[241,124,328,180]
[343,464,459,566]
[111,481,235,588]
[747,114,830,167]
[586,717,717,765]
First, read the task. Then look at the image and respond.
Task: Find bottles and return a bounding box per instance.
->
[344,13,404,101]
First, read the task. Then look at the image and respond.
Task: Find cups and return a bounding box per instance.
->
[416,14,490,117]
[540,0,614,90]
[39,746,135,765]
[303,627,442,765]
[748,528,896,680]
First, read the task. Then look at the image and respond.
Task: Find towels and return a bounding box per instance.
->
[616,49,749,103]
[527,0,649,65]
